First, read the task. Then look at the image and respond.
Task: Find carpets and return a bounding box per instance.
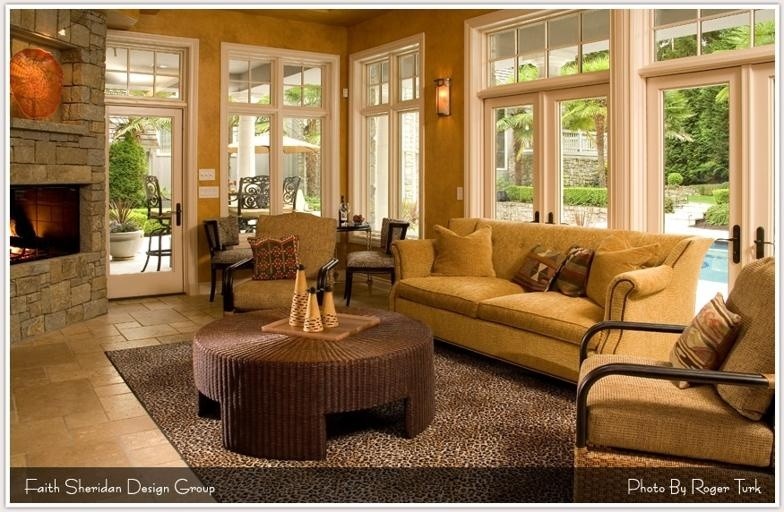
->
[102,342,585,502]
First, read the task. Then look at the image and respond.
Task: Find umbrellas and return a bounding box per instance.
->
[226,130,320,155]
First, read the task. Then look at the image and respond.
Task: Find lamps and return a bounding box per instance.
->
[433,76,453,119]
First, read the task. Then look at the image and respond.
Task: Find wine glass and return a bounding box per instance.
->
[340,203,350,225]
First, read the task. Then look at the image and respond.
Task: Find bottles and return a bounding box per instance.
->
[303,288,325,332]
[337,193,349,224]
[288,265,312,325]
[321,287,340,328]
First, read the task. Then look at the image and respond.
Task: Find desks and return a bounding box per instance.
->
[330,223,374,287]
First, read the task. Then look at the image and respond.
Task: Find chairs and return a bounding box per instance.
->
[576,257,772,496]
[226,174,274,220]
[142,174,175,271]
[223,212,343,316]
[283,176,304,211]
[344,216,413,303]
[204,218,256,302]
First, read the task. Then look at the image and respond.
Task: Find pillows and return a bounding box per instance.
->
[246,232,304,280]
[583,242,661,310]
[555,241,592,297]
[511,241,564,292]
[430,219,497,279]
[671,287,746,386]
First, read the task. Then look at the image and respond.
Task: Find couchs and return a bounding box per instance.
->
[389,217,715,394]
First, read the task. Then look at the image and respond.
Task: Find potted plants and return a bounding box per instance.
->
[107,139,151,261]
